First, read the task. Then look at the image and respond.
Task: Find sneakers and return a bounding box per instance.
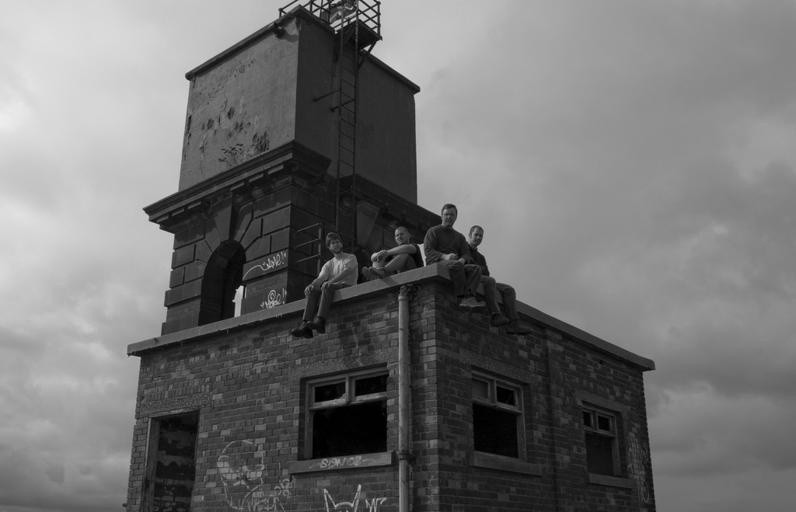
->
[361,267,389,278]
[458,297,486,310]
[489,316,511,328]
[289,320,326,340]
[508,326,535,335]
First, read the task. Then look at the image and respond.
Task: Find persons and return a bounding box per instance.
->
[360,221,424,282]
[423,202,486,308]
[286,230,361,338]
[464,223,536,335]
